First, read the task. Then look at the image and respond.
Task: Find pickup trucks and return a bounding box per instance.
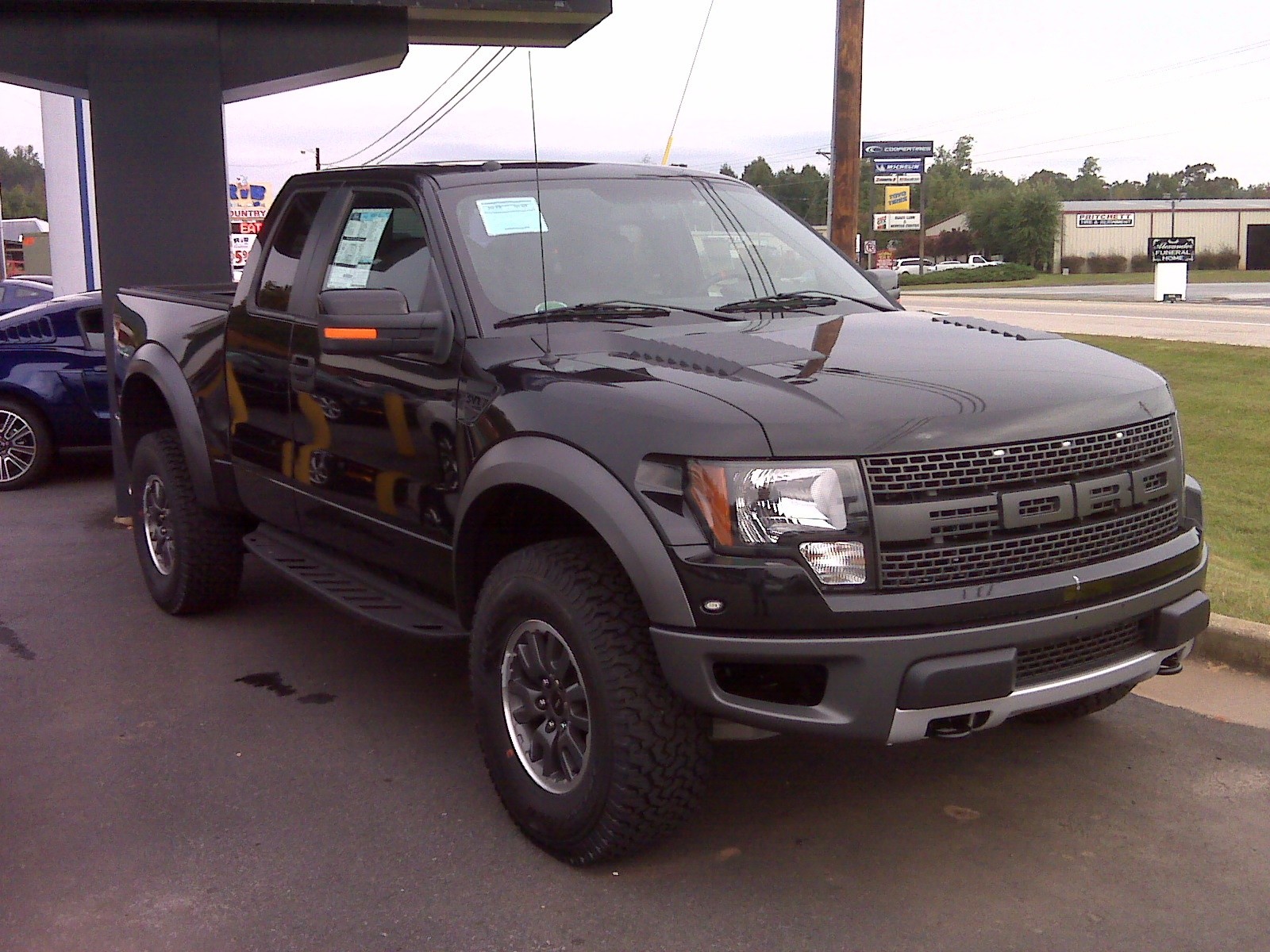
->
[934,255,998,272]
[115,161,1210,869]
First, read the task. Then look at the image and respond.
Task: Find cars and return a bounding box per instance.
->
[0,279,54,317]
[10,275,52,285]
[0,291,136,491]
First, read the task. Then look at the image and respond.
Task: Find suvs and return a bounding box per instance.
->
[892,258,938,276]
